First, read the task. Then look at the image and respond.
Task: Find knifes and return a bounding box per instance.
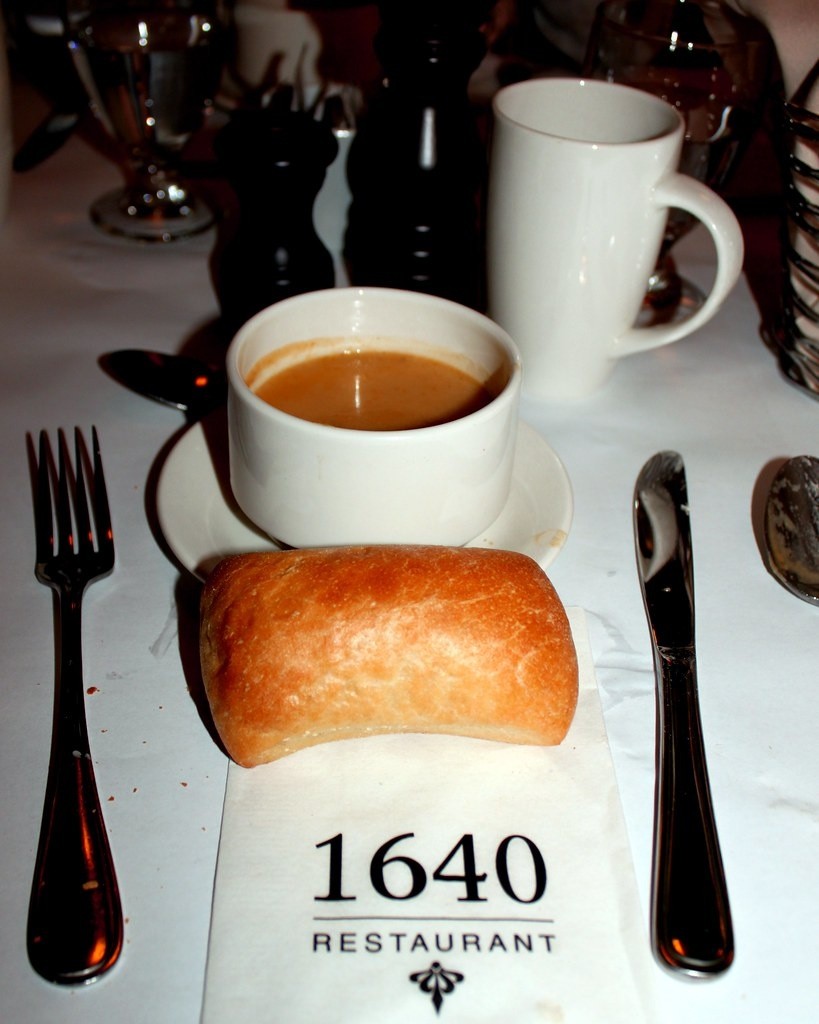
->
[632,450,736,974]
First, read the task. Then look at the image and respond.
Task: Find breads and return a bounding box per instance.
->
[190,541,576,766]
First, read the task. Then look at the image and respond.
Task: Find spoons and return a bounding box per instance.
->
[764,454,819,605]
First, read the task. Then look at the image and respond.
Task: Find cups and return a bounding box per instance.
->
[218,286,530,556]
[481,75,745,370]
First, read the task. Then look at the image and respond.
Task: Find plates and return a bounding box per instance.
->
[156,414,582,597]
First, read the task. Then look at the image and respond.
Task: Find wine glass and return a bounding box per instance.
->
[67,0,219,242]
[582,1,770,332]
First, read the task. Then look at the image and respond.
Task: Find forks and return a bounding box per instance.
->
[22,427,125,985]
